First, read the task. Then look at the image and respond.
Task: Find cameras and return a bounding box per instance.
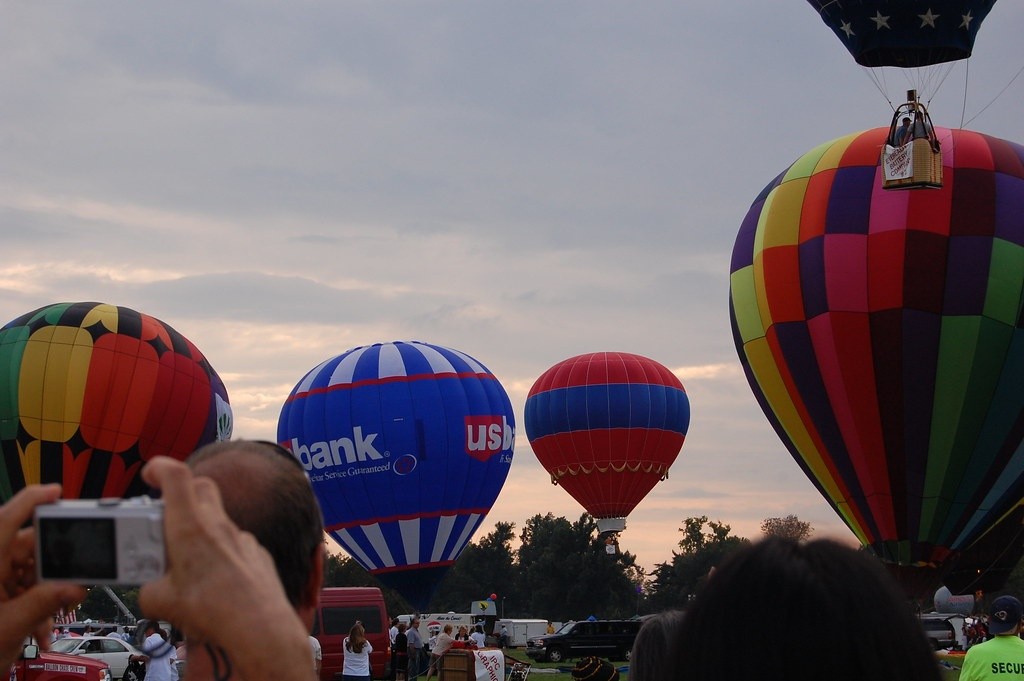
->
[34,493,170,585]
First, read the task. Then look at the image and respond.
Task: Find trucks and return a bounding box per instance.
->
[394,614,550,650]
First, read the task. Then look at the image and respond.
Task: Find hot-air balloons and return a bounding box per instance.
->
[276,340,517,617]
[524,353,691,555]
[730,125,1024,612]
[806,1,996,193]
[0,302,232,532]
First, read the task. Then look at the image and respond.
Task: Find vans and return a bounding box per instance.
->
[916,613,968,653]
[311,587,394,681]
[55,623,137,642]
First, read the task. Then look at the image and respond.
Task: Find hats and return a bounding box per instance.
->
[572,656,619,681]
[989,595,1021,634]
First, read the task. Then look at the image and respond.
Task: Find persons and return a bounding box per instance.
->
[454,625,471,647]
[500,623,509,650]
[121,626,136,646]
[469,623,486,649]
[0,456,327,681]
[571,656,619,681]
[624,538,944,681]
[143,620,181,681]
[404,616,424,681]
[51,627,61,644]
[958,594,1024,680]
[82,625,104,651]
[893,117,912,148]
[341,623,374,681]
[103,626,125,652]
[546,619,554,634]
[394,624,408,681]
[388,616,400,681]
[902,110,936,147]
[63,629,73,639]
[426,623,471,681]
[178,441,328,681]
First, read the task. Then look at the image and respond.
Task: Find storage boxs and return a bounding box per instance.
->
[441,650,505,681]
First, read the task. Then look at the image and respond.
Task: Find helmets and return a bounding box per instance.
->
[548,621,552,624]
[502,624,505,626]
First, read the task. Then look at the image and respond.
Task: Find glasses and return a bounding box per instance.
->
[626,651,631,661]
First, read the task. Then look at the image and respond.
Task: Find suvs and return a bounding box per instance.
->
[525,618,646,664]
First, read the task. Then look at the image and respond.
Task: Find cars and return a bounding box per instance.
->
[48,636,149,681]
[11,644,113,681]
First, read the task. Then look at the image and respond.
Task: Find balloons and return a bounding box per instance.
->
[487,593,497,601]
